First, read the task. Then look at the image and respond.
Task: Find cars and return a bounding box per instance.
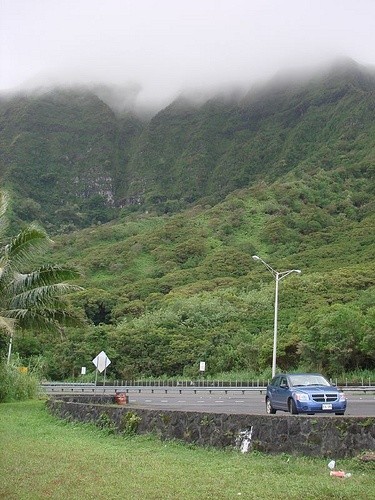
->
[265,373,347,416]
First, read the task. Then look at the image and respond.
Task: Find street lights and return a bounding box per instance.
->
[252,256,302,380]
